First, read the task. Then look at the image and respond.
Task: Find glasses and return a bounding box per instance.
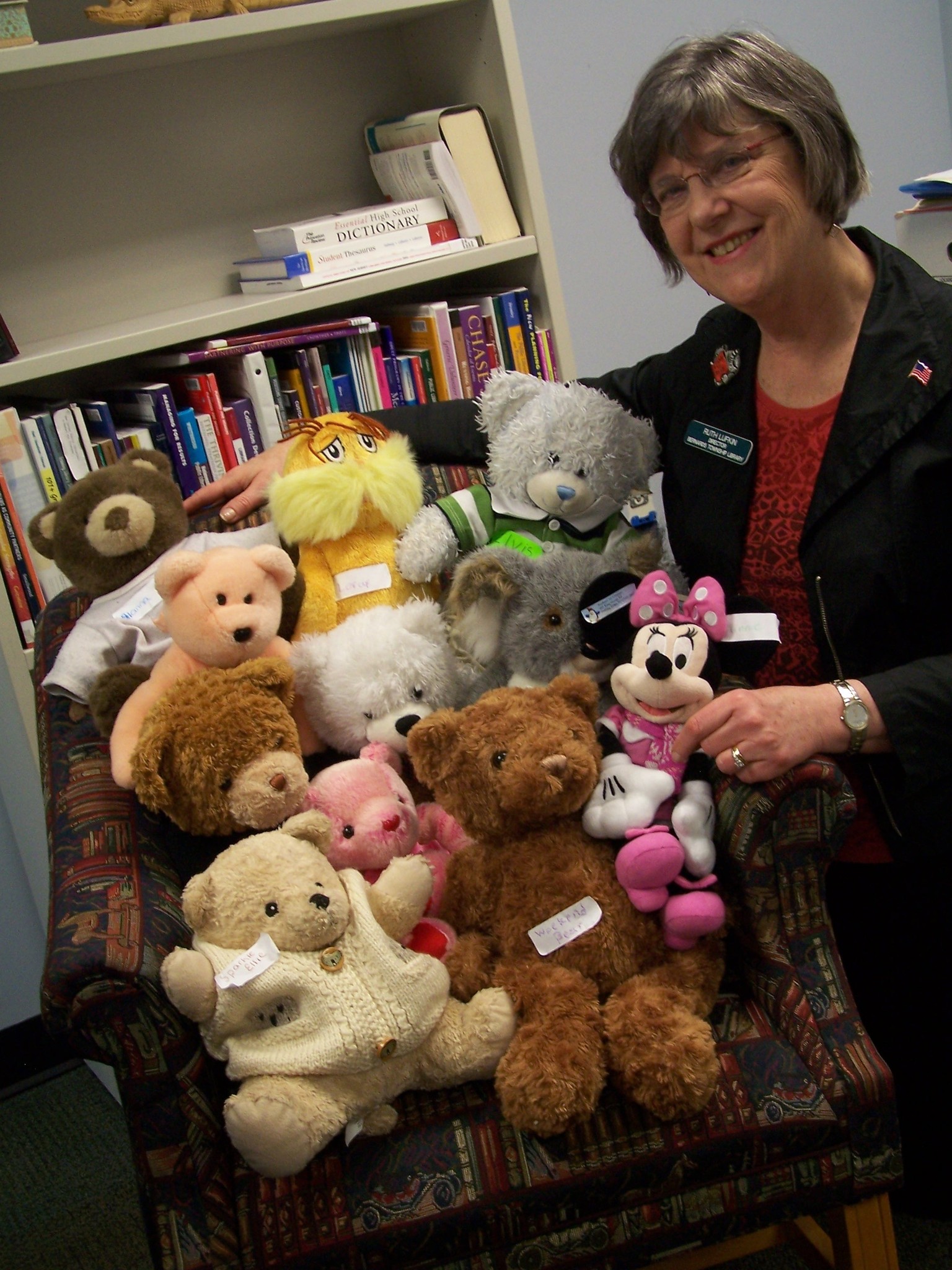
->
[642,125,792,218]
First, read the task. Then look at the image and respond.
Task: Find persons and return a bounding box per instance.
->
[184,27,952,1221]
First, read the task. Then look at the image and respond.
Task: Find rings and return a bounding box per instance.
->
[731,745,746,768]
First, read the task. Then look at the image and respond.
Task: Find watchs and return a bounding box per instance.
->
[831,679,870,755]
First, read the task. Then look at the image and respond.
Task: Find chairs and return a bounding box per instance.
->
[31,465,908,1270]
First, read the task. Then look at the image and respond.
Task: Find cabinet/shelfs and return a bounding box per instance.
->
[0,0,578,943]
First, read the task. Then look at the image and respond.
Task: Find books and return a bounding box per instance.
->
[0,289,560,653]
[229,101,524,293]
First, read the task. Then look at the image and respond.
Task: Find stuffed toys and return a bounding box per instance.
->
[30,374,781,1171]
